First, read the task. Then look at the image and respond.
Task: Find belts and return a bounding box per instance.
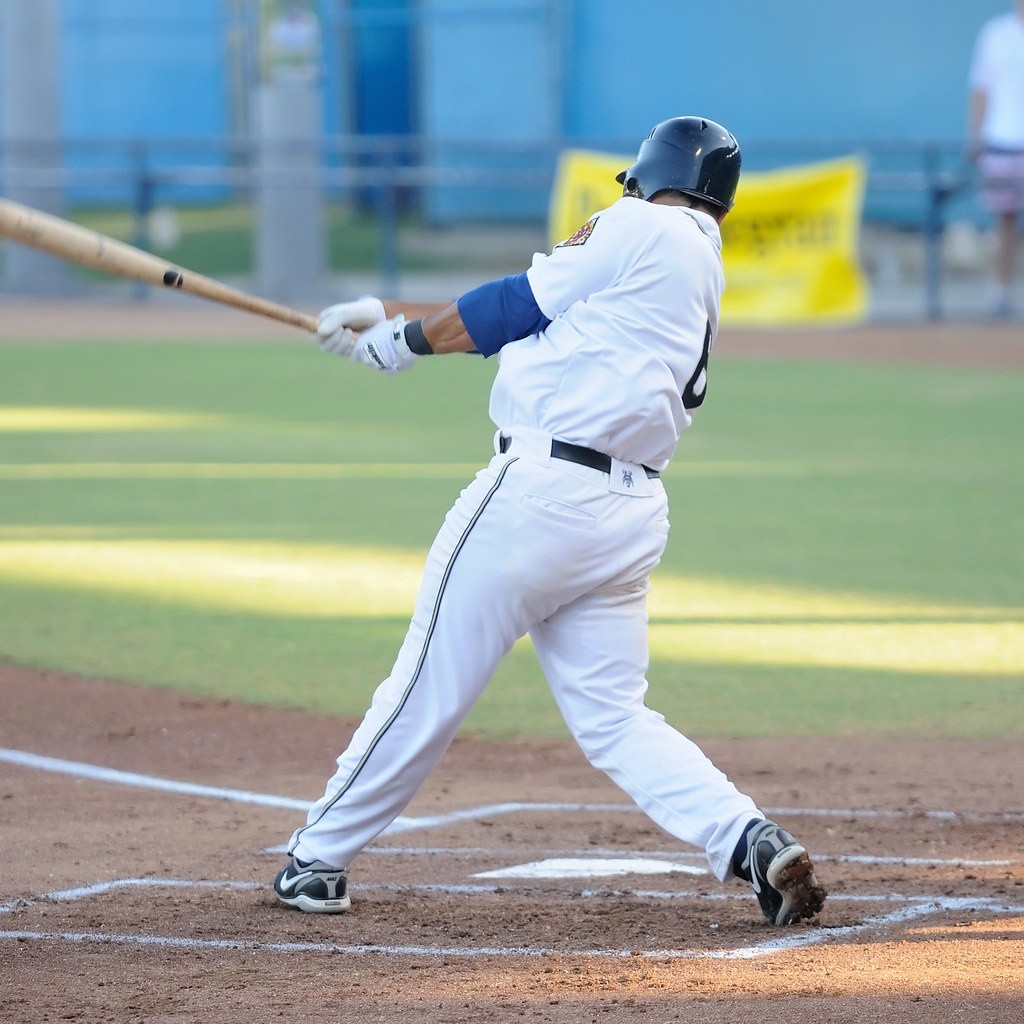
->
[500,436,659,478]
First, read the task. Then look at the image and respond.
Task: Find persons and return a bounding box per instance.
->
[273,116,828,927]
[965,0,1023,323]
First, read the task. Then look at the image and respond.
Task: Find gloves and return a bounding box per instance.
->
[318,298,386,357]
[353,313,419,376]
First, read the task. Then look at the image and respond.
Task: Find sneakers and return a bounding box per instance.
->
[273,856,351,912]
[740,819,826,926]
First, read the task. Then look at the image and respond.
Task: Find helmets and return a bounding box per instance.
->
[615,116,741,213]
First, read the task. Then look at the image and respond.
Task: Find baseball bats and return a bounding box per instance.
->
[0,192,366,350]
